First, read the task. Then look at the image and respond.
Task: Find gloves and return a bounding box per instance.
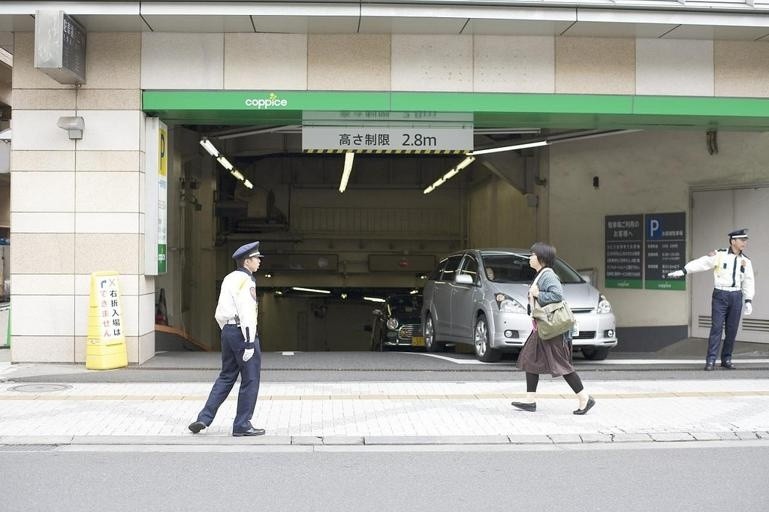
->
[668,270,685,277]
[242,348,254,362]
[743,302,752,316]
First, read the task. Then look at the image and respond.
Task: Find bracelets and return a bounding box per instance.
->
[681,267,688,277]
[744,299,752,304]
[243,341,255,350]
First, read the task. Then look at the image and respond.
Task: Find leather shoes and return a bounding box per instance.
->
[188,420,206,434]
[232,426,266,436]
[704,362,715,371]
[573,395,596,415]
[720,360,735,369]
[511,401,537,412]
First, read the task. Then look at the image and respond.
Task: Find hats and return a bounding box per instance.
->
[728,228,749,239]
[231,240,265,259]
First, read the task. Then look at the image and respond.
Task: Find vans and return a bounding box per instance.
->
[412,244,620,363]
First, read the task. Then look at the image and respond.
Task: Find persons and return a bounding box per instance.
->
[510,240,596,417]
[666,226,756,372]
[188,238,268,438]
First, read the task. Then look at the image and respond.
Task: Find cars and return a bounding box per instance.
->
[365,290,457,354]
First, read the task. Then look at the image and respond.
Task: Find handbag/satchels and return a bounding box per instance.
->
[531,297,576,340]
